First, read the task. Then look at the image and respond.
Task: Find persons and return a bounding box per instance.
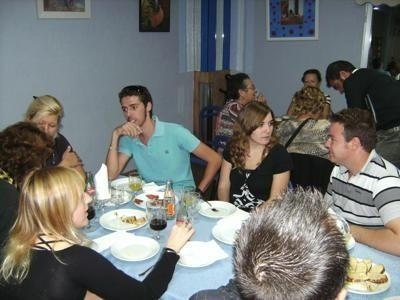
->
[287,69,331,119]
[323,108,400,257]
[326,61,400,169]
[0,166,194,300]
[275,86,336,160]
[212,73,266,182]
[0,122,55,247]
[25,95,86,183]
[372,58,400,81]
[106,86,222,198]
[189,184,350,300]
[217,101,294,215]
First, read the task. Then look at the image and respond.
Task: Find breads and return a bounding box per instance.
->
[347,256,388,283]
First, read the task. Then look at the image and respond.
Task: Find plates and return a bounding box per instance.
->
[110,234,160,263]
[176,242,220,268]
[344,258,392,296]
[340,233,358,252]
[83,170,238,232]
[211,216,250,244]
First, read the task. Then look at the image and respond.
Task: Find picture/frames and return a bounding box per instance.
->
[36,0,91,19]
[266,0,320,42]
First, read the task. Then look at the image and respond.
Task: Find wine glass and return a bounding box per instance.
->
[144,199,168,242]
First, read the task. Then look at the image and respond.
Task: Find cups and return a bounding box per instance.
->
[329,213,350,237]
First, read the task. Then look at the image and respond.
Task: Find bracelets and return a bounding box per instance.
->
[195,188,203,196]
[163,248,176,254]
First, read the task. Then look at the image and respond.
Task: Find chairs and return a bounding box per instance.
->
[190,105,223,166]
